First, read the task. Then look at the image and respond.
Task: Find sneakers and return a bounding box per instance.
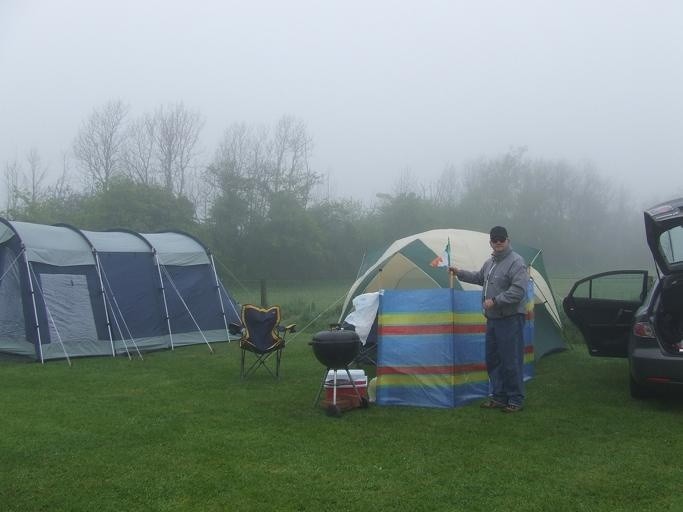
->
[480,400,500,408]
[504,405,521,412]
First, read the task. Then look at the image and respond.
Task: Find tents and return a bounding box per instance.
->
[0,217,249,362]
[335,228,574,409]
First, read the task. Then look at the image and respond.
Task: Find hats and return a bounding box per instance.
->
[490,226,507,240]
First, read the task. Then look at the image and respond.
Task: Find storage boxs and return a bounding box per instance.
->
[324,369,368,407]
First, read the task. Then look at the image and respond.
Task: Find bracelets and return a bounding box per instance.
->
[491,295,497,307]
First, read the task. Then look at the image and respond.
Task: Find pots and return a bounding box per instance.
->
[308,324,363,369]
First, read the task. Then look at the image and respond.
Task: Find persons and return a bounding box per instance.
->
[447,226,530,413]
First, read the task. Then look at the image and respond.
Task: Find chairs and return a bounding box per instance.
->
[228,304,297,381]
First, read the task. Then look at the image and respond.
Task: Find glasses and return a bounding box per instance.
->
[492,239,506,243]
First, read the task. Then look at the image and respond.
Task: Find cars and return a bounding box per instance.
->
[562,197,683,397]
